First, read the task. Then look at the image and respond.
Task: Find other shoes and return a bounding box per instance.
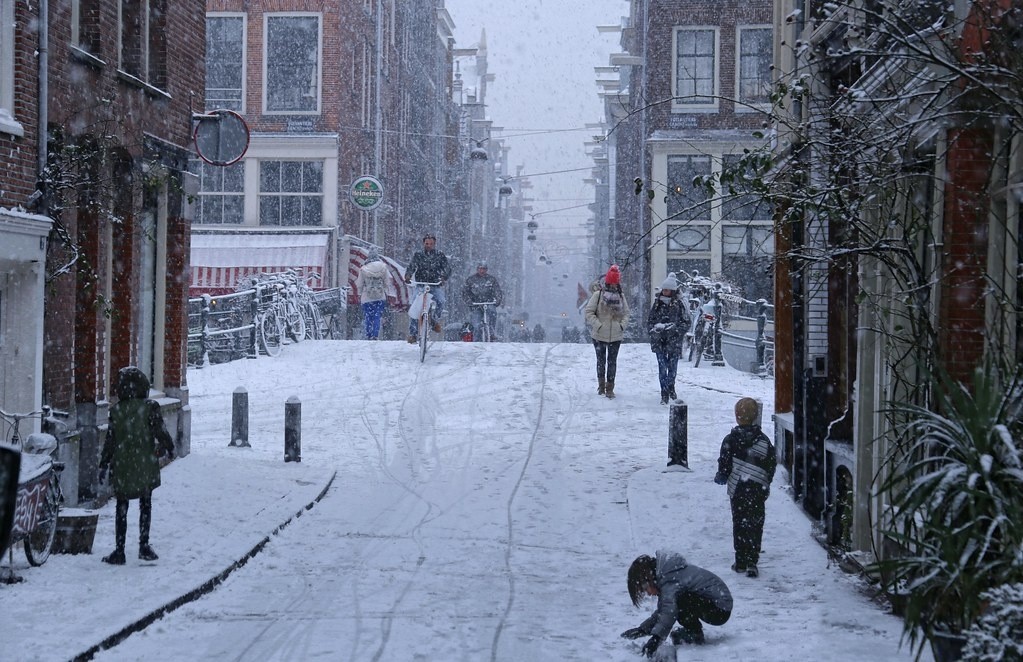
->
[670,627,705,645]
[408,335,418,344]
[139,544,159,561]
[669,386,677,400]
[431,324,441,333]
[660,392,669,404]
[101,550,126,564]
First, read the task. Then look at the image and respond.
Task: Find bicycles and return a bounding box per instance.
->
[408,280,443,363]
[473,302,496,341]
[0,404,70,570]
[259,271,322,358]
[675,269,731,368]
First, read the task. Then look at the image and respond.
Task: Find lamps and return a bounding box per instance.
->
[526,230,536,240]
[539,251,547,262]
[25,190,43,213]
[469,137,489,164]
[553,274,558,280]
[545,256,552,265]
[562,273,569,279]
[558,281,564,286]
[527,213,538,231]
[498,176,513,196]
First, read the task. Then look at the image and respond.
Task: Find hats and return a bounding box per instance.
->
[605,265,621,284]
[365,245,379,263]
[661,272,678,290]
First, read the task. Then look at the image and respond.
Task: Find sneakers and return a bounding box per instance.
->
[731,562,746,573]
[746,563,758,577]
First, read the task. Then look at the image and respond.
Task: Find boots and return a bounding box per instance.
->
[597,377,605,395]
[605,382,614,398]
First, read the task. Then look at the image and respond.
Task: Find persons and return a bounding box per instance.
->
[509,324,544,342]
[620,549,733,659]
[405,234,452,343]
[562,325,591,343]
[356,244,393,340]
[98,366,174,565]
[714,398,778,577]
[584,265,630,399]
[462,260,503,342]
[647,272,692,405]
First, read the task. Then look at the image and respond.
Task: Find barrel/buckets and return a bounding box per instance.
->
[29,507,100,555]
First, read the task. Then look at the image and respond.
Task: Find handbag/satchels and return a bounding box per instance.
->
[363,265,387,299]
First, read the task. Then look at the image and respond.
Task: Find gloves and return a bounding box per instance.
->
[642,635,662,658]
[169,450,177,461]
[620,627,647,640]
[99,469,106,485]
